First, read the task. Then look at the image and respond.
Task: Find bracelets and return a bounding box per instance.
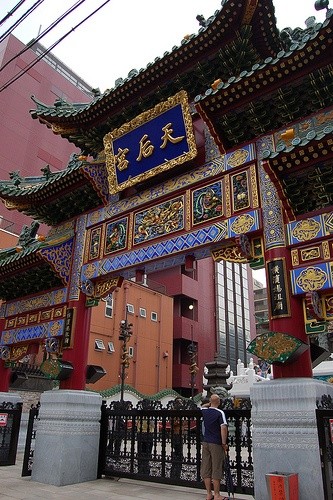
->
[222,444,226,446]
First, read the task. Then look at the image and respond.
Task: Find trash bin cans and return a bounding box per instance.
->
[263,470,300,500]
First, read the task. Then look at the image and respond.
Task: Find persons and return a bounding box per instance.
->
[200,394,229,500]
[137,400,155,475]
[169,397,184,456]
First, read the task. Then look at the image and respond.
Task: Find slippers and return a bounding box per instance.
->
[207,495,214,500]
[222,497,229,500]
